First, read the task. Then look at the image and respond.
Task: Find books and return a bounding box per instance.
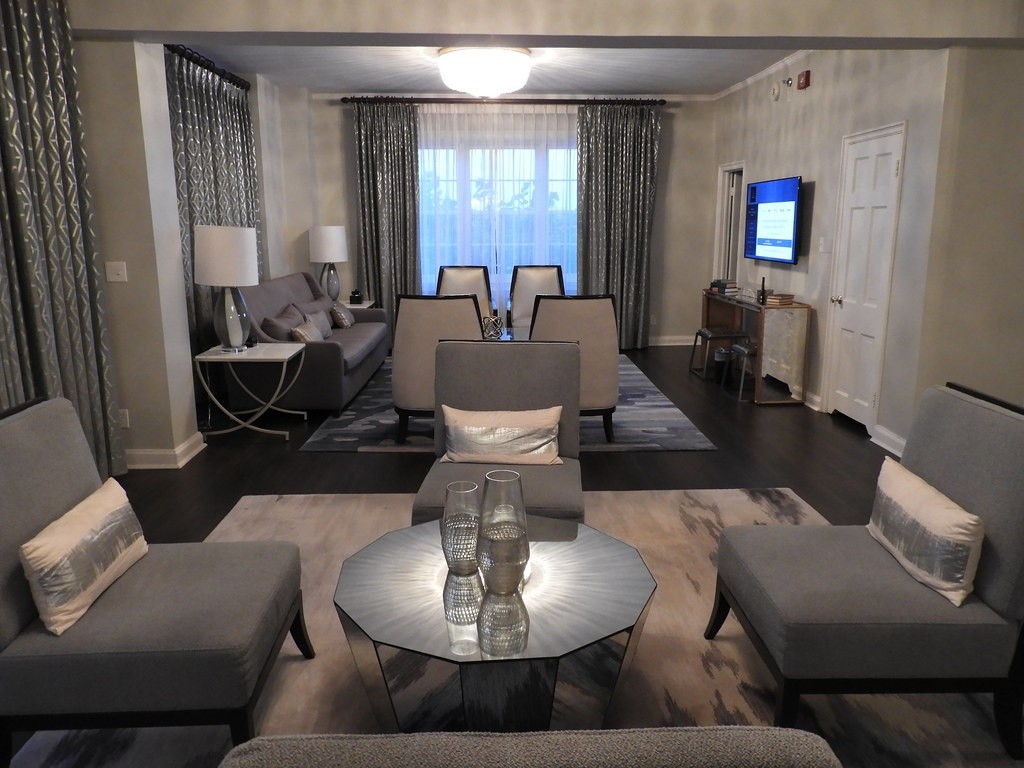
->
[711,279,742,296]
[766,294,795,305]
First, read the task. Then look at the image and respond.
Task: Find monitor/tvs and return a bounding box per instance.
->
[744,175,802,264]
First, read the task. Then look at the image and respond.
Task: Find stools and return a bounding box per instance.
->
[688,325,750,381]
[720,345,757,403]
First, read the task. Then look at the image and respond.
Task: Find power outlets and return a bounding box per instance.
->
[117,409,130,430]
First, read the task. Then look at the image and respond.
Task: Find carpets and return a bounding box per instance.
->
[298,356,721,452]
[12,491,1024,768]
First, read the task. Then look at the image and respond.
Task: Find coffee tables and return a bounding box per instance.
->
[331,508,658,730]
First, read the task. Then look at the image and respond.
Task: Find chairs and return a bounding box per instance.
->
[390,265,618,442]
[410,339,586,526]
[703,379,1024,760]
[1,396,317,752]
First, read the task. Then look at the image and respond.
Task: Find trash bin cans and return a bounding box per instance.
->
[713,349,734,385]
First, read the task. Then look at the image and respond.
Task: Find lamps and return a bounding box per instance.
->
[192,224,261,350]
[308,224,348,301]
[437,45,532,99]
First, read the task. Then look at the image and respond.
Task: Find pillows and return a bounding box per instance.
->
[333,301,356,328]
[259,304,305,340]
[291,294,334,332]
[17,478,149,635]
[439,401,565,465]
[304,310,333,338]
[865,455,986,607]
[292,319,324,343]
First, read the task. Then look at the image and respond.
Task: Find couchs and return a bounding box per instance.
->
[210,272,389,420]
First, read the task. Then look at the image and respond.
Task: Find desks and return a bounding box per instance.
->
[194,340,307,442]
[483,328,531,343]
[698,288,812,405]
[338,300,375,309]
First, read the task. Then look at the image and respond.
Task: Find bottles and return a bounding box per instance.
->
[757,276,767,305]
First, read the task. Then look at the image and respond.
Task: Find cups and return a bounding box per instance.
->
[476,591,531,660]
[441,479,481,576]
[475,468,530,596]
[442,569,486,626]
